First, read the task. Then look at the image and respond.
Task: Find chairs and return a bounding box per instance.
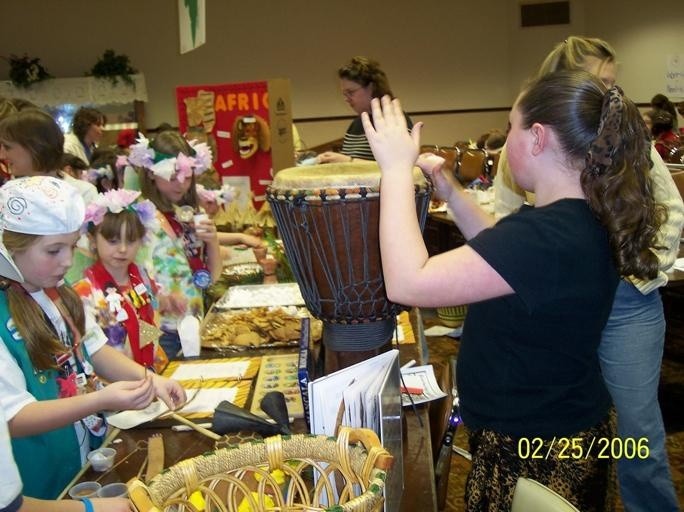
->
[419,142,502,188]
[429,355,461,512]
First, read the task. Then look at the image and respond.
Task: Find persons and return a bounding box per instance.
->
[359,71,670,512]
[0,96,262,512]
[316,55,412,164]
[650,94,684,134]
[648,110,684,163]
[492,34,684,512]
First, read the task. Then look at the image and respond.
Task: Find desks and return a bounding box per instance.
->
[51,271,440,512]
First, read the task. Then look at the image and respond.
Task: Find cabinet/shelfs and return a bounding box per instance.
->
[0,71,149,160]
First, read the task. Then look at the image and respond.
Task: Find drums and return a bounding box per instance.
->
[265,160,435,376]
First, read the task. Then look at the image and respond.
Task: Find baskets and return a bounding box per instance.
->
[127,425,392,512]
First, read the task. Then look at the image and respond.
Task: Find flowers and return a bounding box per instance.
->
[78,127,213,239]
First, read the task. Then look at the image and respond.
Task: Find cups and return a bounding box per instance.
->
[190,213,209,236]
[65,481,128,502]
[87,446,117,472]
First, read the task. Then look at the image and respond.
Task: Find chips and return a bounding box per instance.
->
[201,307,301,350]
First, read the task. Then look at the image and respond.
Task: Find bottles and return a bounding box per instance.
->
[175,302,203,359]
